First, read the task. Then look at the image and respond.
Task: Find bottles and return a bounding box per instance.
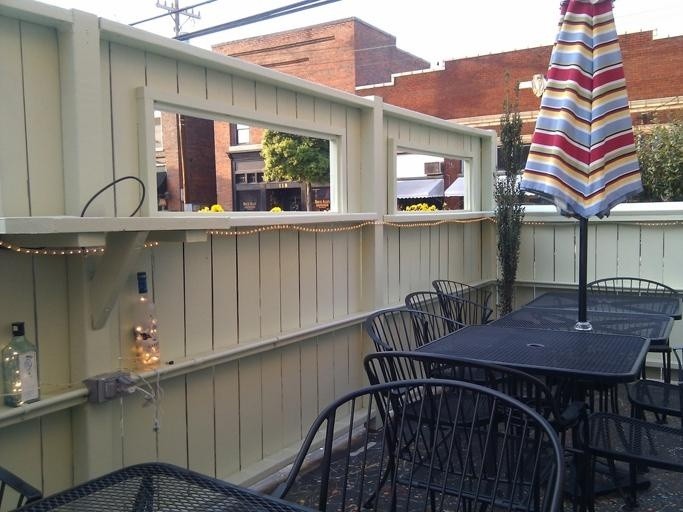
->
[1,322,41,408]
[129,271,161,371]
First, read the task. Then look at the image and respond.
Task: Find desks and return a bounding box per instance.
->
[8,459,316,512]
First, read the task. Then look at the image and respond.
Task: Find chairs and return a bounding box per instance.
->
[586,276,678,296]
[0,466,42,511]
[405,291,506,385]
[268,378,565,512]
[364,351,592,511]
[432,279,493,324]
[364,308,499,472]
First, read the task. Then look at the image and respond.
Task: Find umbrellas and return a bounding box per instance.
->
[519,1,643,322]
